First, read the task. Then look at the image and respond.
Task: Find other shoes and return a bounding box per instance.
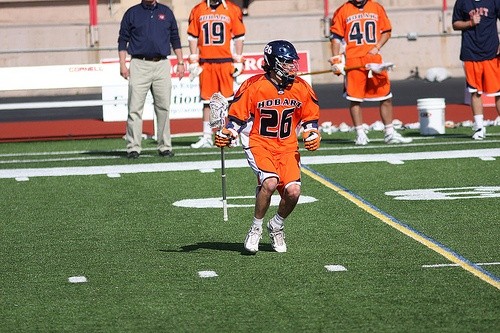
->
[471,127,487,140]
[384,130,412,144]
[159,151,174,157]
[355,133,369,145]
[191,137,214,148]
[128,150,139,158]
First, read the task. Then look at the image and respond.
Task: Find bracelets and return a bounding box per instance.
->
[469,20,472,27]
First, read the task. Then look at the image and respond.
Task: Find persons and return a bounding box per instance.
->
[328,0,413,144]
[118,0,184,158]
[451,0,500,140]
[188,0,246,149]
[214,40,321,256]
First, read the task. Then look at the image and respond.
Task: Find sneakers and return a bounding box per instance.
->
[244,223,263,254]
[267,219,287,252]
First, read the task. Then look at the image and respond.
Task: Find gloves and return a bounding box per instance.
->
[214,120,248,148]
[188,53,203,76]
[231,54,245,77]
[302,122,322,151]
[328,53,346,76]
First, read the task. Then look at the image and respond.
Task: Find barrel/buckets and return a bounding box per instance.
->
[417,98,445,134]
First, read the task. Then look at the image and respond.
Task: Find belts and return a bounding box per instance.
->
[131,55,168,62]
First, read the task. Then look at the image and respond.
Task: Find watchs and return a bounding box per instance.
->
[177,62,183,64]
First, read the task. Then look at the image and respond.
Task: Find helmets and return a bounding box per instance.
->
[264,40,300,88]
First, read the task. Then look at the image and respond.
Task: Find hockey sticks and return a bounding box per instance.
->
[299,62,395,77]
[208,91,230,223]
